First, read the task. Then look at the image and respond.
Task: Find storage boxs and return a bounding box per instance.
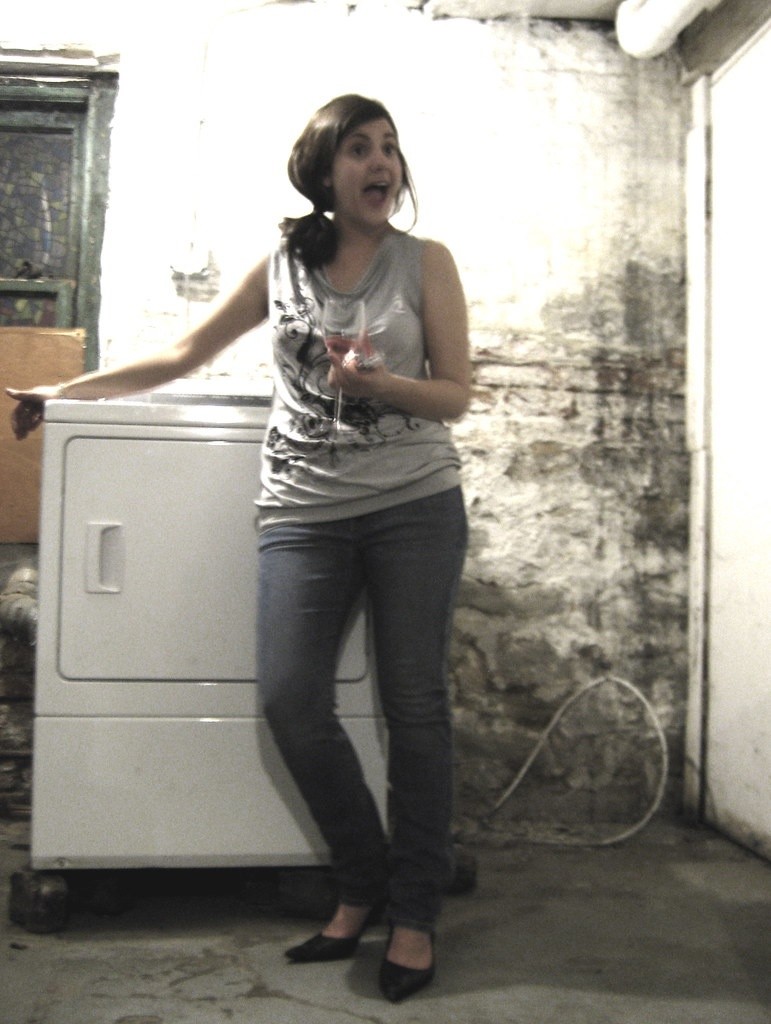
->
[0,325,87,543]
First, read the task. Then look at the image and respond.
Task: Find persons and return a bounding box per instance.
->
[3,94,474,1009]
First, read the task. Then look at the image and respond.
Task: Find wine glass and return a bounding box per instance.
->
[317,296,372,434]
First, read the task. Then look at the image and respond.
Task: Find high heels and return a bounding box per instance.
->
[284,889,393,961]
[379,922,436,1002]
